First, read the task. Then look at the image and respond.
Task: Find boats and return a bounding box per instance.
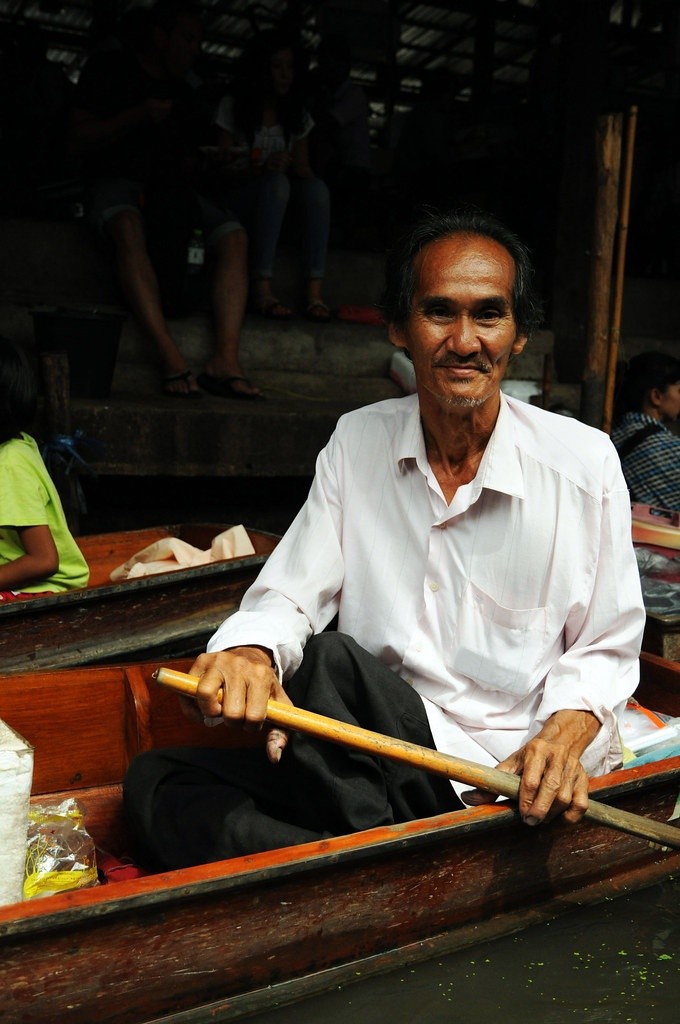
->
[1,520,289,676]
[0,647,680,1024]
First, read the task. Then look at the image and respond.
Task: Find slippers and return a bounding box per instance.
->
[201,370,266,400]
[159,372,201,398]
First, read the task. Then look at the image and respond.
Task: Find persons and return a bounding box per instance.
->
[0,334,88,601]
[612,365,680,511]
[212,33,332,320]
[124,215,646,877]
[72,0,265,400]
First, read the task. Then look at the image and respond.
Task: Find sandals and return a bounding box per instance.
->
[262,300,298,320]
[306,299,329,324]
